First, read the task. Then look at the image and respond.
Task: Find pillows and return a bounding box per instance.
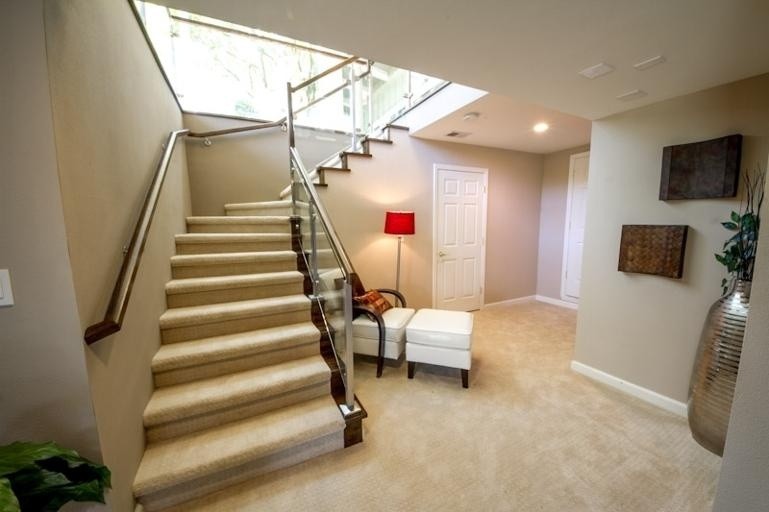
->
[352,290,392,322]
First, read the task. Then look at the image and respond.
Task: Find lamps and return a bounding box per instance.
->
[385,212,416,307]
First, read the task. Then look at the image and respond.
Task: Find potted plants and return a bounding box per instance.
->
[688,165,766,458]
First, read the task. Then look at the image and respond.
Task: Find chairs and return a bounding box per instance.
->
[344,275,415,378]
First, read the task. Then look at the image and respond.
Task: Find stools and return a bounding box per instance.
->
[405,308,475,388]
